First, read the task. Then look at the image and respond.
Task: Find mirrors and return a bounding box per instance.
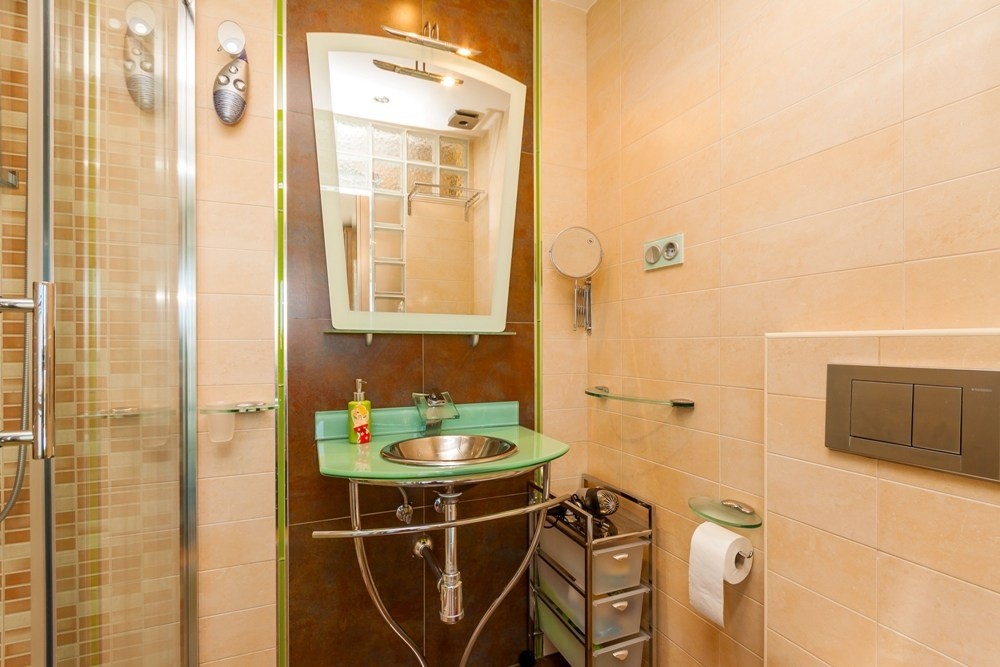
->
[307,31,526,335]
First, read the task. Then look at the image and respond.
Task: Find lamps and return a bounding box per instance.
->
[383,23,480,56]
[214,20,249,124]
[373,58,462,85]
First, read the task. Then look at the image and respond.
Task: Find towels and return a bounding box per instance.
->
[689,521,754,628]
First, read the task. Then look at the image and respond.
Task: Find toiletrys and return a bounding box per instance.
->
[348,379,371,444]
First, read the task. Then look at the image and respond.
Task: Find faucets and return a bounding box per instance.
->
[412,391,460,437]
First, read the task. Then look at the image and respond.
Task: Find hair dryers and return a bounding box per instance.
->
[537,486,619,529]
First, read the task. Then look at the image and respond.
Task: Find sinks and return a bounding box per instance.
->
[380,434,518,467]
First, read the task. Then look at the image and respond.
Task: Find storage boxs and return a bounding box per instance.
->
[539,519,651,596]
[537,555,650,644]
[536,593,650,667]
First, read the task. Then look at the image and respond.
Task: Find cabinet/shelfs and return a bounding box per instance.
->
[525,474,659,667]
